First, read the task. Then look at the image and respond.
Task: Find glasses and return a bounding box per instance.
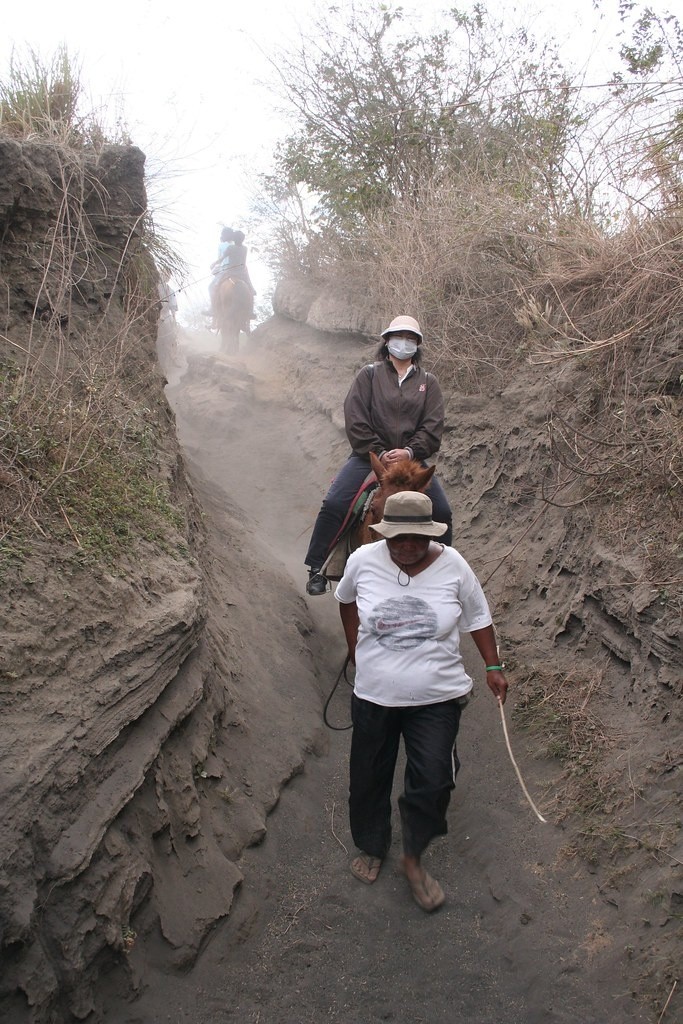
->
[388,533,431,545]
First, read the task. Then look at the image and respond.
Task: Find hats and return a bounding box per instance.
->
[381,315,423,347]
[368,490,448,538]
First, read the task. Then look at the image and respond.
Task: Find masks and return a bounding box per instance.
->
[386,335,419,360]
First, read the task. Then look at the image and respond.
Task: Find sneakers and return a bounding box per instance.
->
[306,567,328,595]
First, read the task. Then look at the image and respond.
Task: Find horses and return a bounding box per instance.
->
[214,274,254,356]
[338,451,438,669]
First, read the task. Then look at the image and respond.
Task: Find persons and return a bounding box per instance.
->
[201,228,258,319]
[304,316,453,595]
[333,492,508,912]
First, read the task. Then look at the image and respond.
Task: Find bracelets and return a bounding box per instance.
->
[486,666,502,671]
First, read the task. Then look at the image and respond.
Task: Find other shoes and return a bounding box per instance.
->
[201,308,215,316]
[249,313,256,319]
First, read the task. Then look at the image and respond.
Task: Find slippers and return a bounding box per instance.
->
[350,849,382,884]
[400,853,446,911]
